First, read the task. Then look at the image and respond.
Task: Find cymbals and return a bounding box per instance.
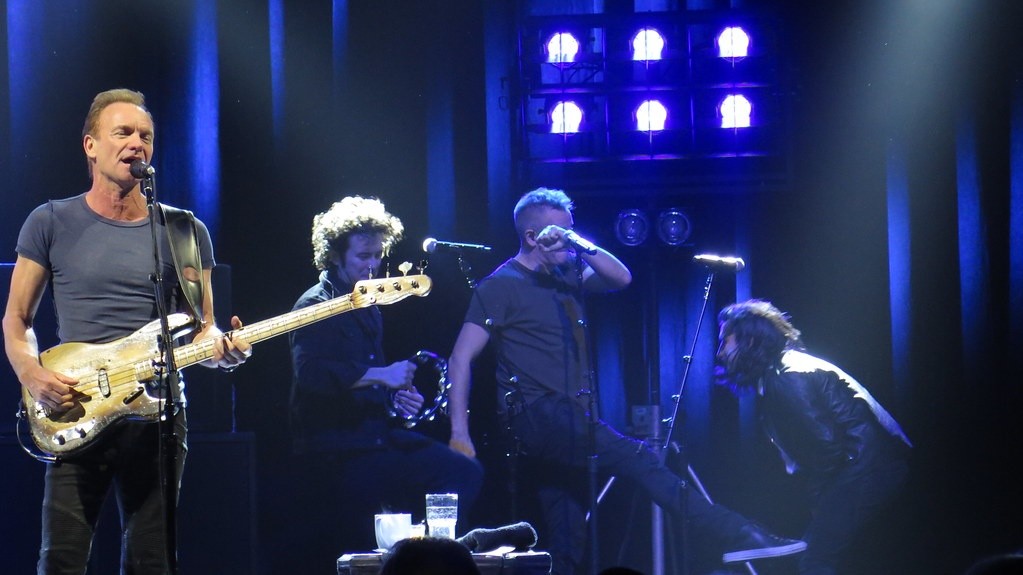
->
[380,350,454,431]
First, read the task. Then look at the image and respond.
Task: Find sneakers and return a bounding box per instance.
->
[722,522,808,565]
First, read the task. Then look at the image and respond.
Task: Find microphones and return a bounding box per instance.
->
[563,230,597,256]
[692,254,746,272]
[423,237,491,255]
[130,161,156,179]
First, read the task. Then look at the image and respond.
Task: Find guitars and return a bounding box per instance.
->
[20,260,435,458]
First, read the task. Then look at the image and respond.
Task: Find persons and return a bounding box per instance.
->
[3,88,253,575]
[378,537,482,575]
[288,196,484,538]
[448,188,807,575]
[716,299,913,575]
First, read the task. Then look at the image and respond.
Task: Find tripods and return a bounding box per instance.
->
[583,272,758,575]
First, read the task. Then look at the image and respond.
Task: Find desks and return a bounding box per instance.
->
[336,552,553,575]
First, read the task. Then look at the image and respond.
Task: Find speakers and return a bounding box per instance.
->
[0,262,258,575]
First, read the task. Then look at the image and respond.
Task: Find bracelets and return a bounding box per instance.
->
[218,364,239,372]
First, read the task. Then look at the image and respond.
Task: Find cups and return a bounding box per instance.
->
[426,494,458,540]
[375,513,425,551]
